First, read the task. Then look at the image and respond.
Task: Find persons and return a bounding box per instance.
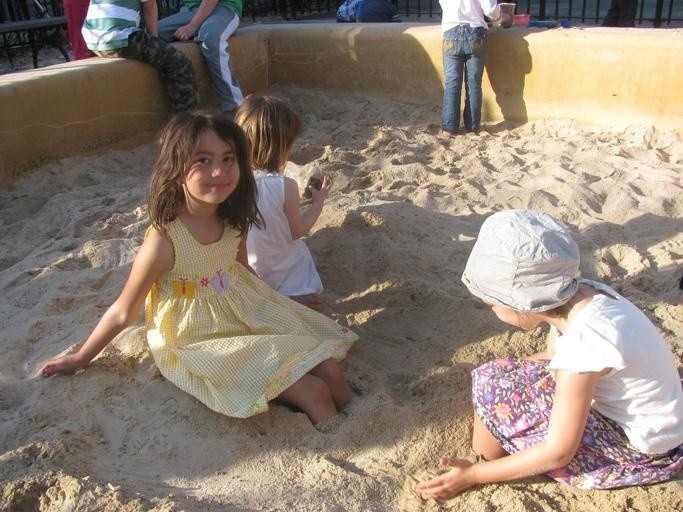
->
[336,1,406,25]
[435,0,512,139]
[81,0,198,111]
[411,206,683,499]
[39,110,358,428]
[155,2,243,113]
[233,96,329,314]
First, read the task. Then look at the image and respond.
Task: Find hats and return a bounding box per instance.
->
[461,209,580,312]
